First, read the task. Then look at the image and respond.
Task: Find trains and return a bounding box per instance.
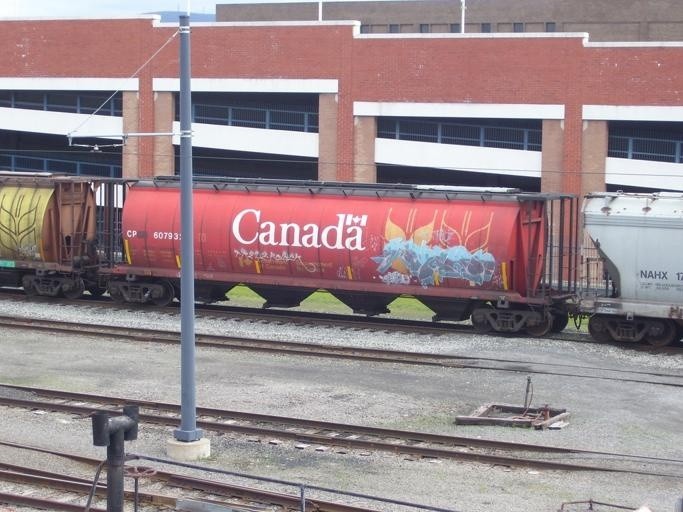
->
[0,170,683,346]
